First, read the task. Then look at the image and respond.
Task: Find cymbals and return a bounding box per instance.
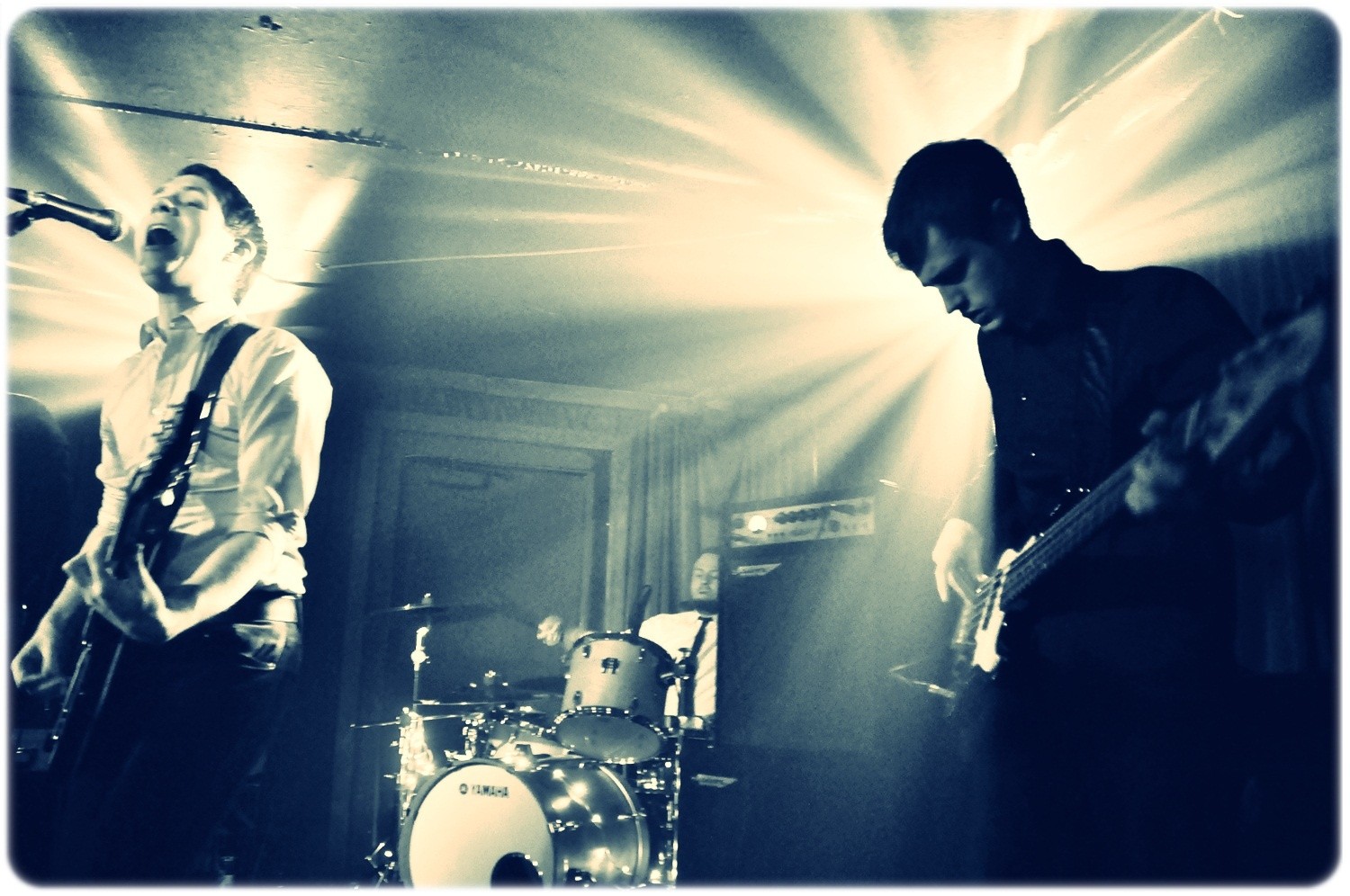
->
[368,599,499,630]
[408,686,564,710]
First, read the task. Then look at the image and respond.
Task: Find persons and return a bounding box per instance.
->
[882,139,1311,881]
[620,547,720,728]
[11,163,333,883]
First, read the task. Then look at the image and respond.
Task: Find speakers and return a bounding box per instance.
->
[714,492,964,724]
[679,728,985,884]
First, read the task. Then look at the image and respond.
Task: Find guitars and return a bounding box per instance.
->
[939,303,1331,727]
[44,172,359,780]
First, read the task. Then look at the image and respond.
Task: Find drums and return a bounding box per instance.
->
[551,628,676,765]
[616,713,712,830]
[487,710,557,742]
[398,755,650,887]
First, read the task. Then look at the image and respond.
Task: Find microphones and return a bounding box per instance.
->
[8,184,130,245]
